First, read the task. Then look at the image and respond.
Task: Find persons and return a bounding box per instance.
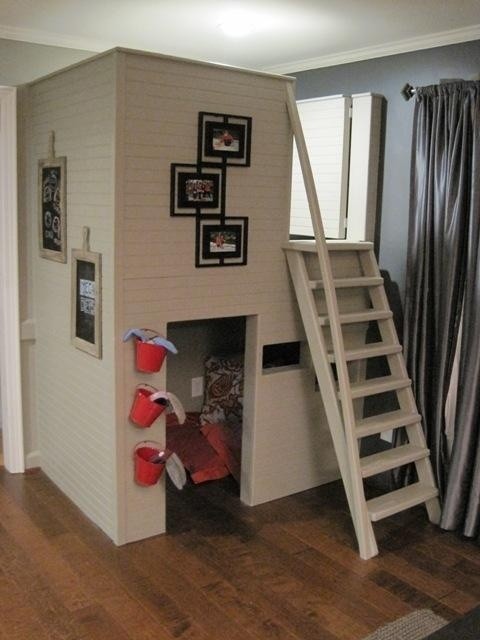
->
[215,234,224,249]
[185,180,211,199]
[215,128,234,145]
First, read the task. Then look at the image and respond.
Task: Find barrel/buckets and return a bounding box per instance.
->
[130,383,169,428]
[135,329,167,373]
[133,439,166,487]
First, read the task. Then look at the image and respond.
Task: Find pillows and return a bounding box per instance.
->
[199,354,244,426]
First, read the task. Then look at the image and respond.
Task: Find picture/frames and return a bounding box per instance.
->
[170,109,253,270]
